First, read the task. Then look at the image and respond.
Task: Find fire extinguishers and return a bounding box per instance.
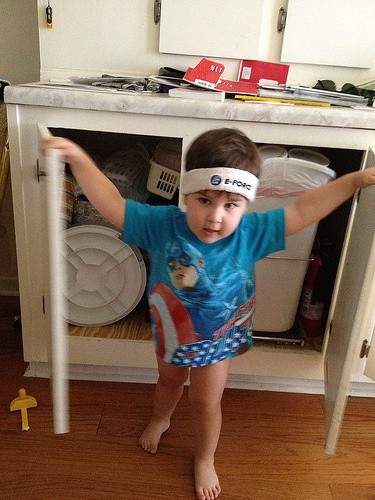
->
[298,240,333,344]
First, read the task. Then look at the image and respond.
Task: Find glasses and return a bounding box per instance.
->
[312,79,358,95]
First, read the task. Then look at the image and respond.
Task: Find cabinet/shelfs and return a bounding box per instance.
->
[6,102,375,454]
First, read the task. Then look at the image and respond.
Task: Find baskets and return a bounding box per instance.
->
[101,147,148,202]
[148,154,180,201]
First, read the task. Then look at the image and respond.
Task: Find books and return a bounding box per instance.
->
[182,59,369,108]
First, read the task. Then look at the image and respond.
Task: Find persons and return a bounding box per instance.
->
[39,127,375,500]
